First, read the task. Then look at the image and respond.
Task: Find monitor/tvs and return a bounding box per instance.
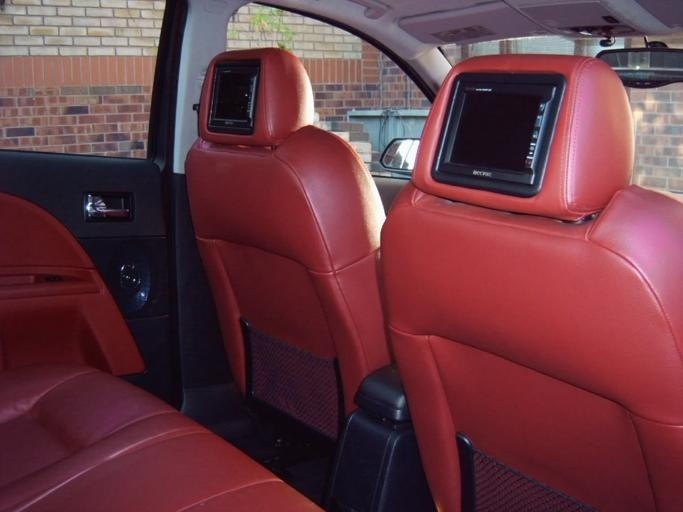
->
[438,84,552,185]
[210,70,258,127]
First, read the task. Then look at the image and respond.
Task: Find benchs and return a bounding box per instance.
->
[1,360,332,512]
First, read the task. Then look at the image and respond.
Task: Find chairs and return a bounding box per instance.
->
[185,44,392,435]
[377,50,682,510]
